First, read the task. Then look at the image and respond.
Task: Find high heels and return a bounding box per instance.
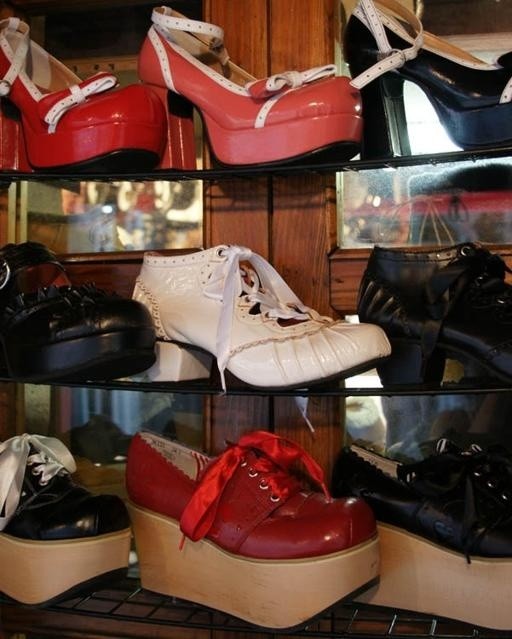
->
[359,242,512,388]
[133,245,391,388]
[345,0,511,160]
[126,430,380,628]
[2,241,154,379]
[139,8,363,169]
[0,17,166,173]
[0,433,132,610]
[331,444,512,630]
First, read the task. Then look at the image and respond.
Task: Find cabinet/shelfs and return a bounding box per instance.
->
[0,147,512,638]
